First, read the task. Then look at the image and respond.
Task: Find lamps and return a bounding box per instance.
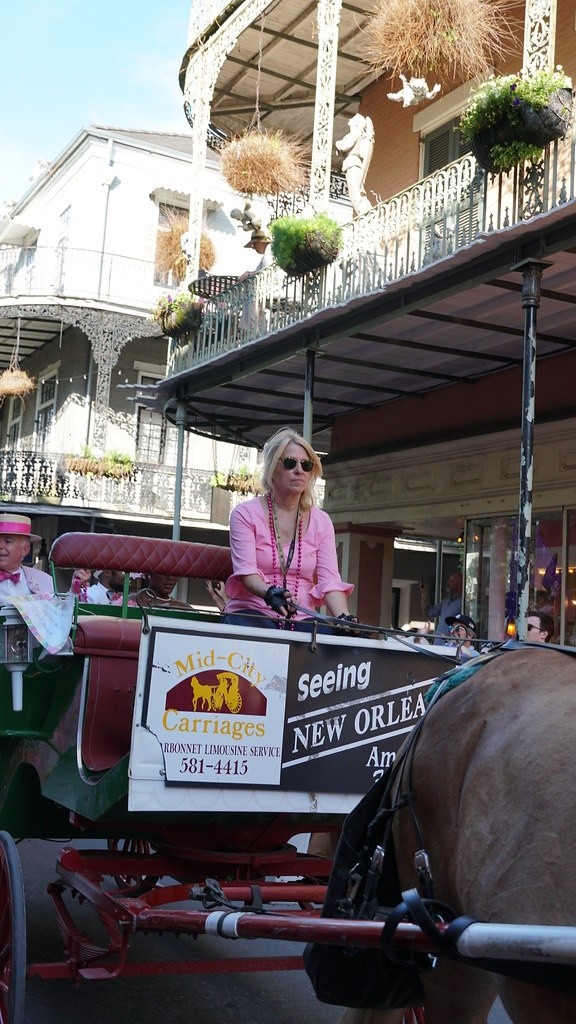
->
[458,531,464,542]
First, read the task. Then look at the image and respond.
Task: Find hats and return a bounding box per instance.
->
[445,615,476,634]
[93,571,103,579]
[0,514,42,542]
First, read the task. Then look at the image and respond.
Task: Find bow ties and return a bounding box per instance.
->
[0,569,20,584]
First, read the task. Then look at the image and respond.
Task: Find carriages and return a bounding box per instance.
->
[0,532,575,1024]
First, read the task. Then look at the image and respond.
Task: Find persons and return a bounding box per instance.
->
[131,573,195,609]
[441,613,480,658]
[523,610,554,644]
[239,230,282,298]
[0,513,92,607]
[81,568,132,607]
[222,426,372,638]
[386,75,442,109]
[229,208,263,233]
[205,580,229,609]
[335,113,375,217]
[428,572,465,647]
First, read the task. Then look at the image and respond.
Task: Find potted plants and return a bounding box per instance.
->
[0,367,38,409]
[158,201,215,277]
[470,88,573,173]
[270,213,344,276]
[220,121,312,197]
[353,0,525,90]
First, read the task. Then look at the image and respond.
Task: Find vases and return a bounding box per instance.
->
[156,302,205,339]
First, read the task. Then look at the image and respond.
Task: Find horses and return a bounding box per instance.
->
[339,647,576,1024]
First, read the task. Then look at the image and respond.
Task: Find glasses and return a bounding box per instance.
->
[528,624,544,631]
[278,457,315,472]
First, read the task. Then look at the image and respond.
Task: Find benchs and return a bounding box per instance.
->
[49,532,234,659]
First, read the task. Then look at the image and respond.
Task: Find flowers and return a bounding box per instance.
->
[149,287,205,320]
[453,65,573,173]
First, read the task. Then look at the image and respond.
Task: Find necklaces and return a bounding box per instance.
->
[73,576,88,603]
[264,493,303,630]
